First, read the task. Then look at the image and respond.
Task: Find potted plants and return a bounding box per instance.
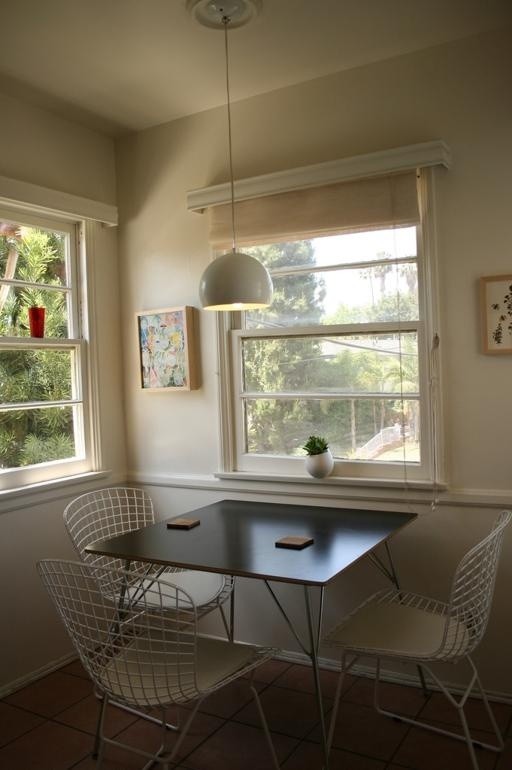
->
[303,435,334,477]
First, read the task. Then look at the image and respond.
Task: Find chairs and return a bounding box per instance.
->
[33,559,279,770]
[318,506,512,770]
[61,487,234,732]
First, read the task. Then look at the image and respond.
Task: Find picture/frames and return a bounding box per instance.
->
[135,305,203,393]
[478,271,512,357]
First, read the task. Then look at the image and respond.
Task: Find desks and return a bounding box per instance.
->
[83,499,430,770]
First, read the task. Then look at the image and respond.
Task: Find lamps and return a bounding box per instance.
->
[182,0,275,312]
